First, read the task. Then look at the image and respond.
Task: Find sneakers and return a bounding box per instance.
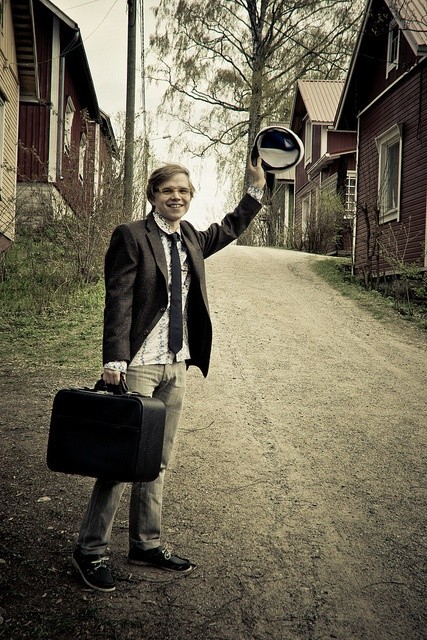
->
[127,544,192,572]
[70,546,115,593]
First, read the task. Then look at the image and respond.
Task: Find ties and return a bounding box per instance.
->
[165,232,183,355]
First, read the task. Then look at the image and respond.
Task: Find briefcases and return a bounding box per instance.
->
[46,374,166,483]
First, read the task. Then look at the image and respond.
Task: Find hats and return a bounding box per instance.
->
[251,126,305,174]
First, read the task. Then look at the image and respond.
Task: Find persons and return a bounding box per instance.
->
[71,153,267,592]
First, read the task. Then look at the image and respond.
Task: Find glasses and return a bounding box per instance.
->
[152,187,190,195]
